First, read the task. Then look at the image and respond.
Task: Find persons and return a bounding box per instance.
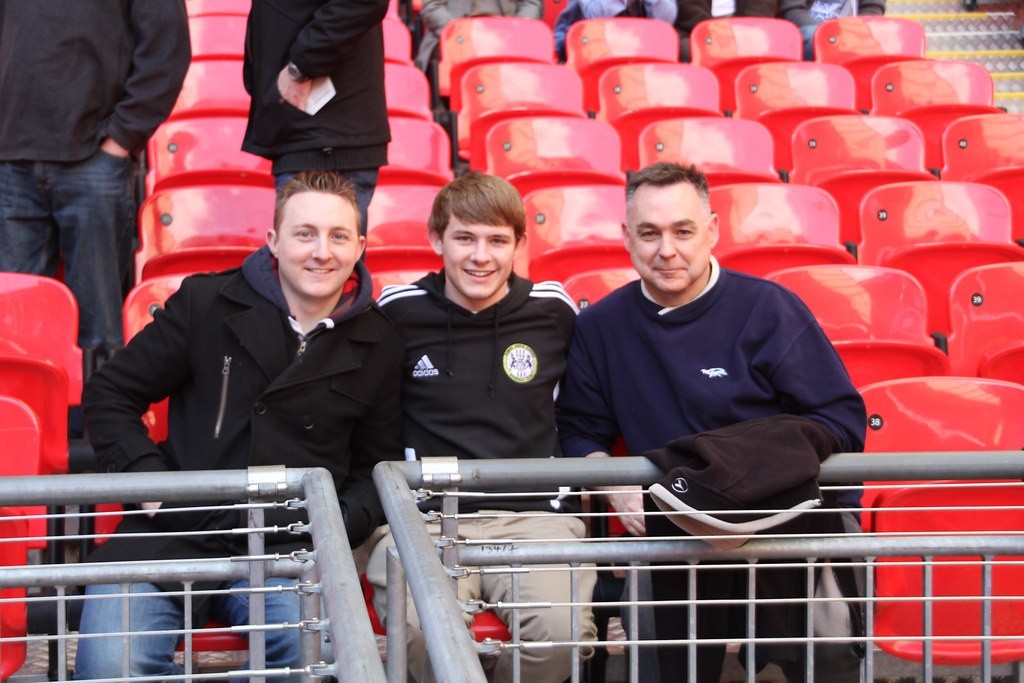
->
[240,0,392,261]
[0,1,193,473]
[414,0,887,72]
[365,170,599,683]
[555,164,867,683]
[74,173,409,683]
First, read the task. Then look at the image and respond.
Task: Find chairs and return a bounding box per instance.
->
[1,0,1024,683]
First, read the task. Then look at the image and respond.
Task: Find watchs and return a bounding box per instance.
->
[287,60,307,83]
